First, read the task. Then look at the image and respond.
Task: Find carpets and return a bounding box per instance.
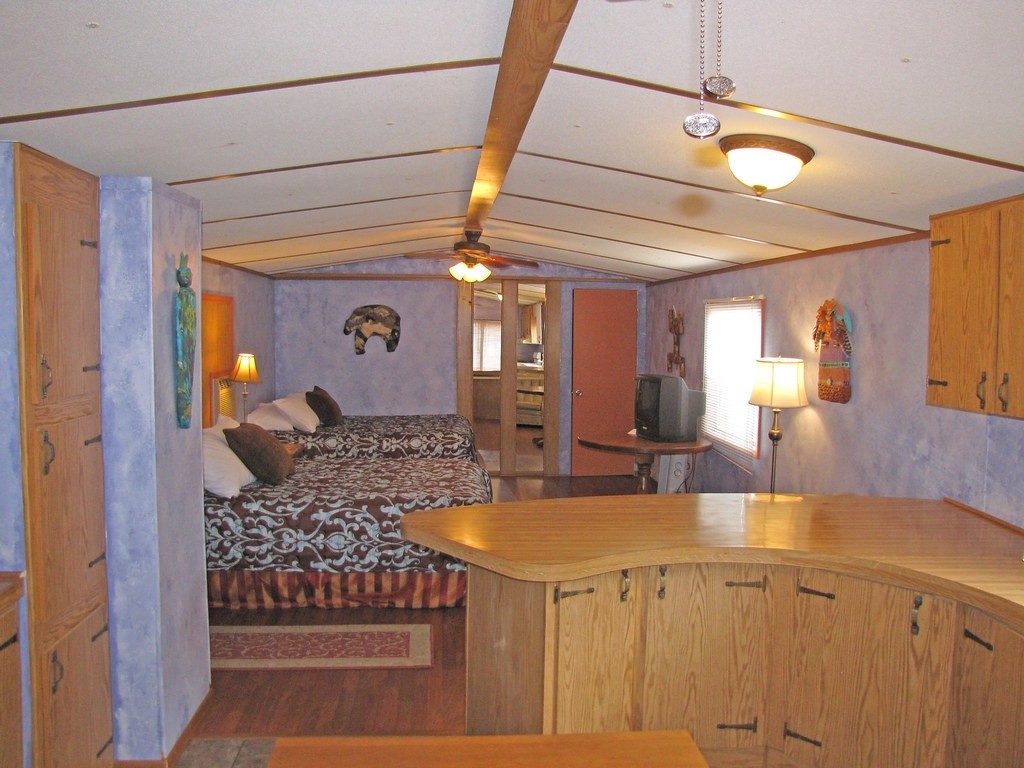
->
[208,623,435,671]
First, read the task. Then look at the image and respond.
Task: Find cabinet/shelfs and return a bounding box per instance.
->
[925,192,1024,420]
[0,146,123,768]
[517,302,545,345]
[399,493,1024,768]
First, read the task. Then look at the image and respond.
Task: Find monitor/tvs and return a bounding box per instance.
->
[635,373,707,443]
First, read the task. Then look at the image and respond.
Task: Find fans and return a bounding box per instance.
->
[402,241,538,283]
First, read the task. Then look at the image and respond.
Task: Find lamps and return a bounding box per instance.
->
[748,358,810,492]
[228,353,259,422]
[718,134,815,197]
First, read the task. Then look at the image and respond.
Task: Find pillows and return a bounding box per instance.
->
[202,414,257,499]
[305,385,342,426]
[247,402,294,432]
[272,391,320,433]
[222,422,296,486]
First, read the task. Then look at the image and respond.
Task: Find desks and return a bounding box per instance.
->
[577,431,713,494]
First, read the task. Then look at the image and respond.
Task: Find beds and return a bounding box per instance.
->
[272,415,480,464]
[204,458,493,609]
